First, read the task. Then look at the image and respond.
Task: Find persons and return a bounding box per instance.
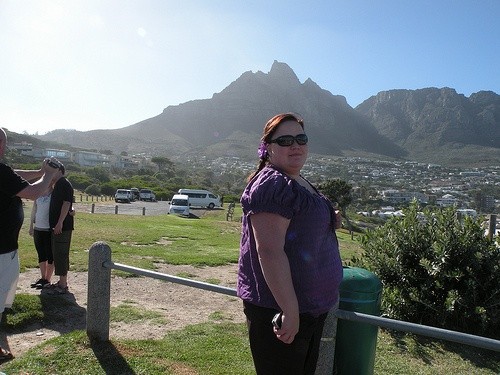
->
[0,127,76,360]
[238,113,344,375]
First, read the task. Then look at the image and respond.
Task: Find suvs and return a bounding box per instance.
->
[138,189,156,202]
[127,187,140,202]
[115,189,131,204]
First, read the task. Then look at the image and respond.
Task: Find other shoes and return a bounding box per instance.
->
[0,349,15,361]
[31,278,51,289]
[46,282,68,295]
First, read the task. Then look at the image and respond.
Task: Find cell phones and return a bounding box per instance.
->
[272,313,283,331]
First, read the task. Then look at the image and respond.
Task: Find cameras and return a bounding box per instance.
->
[45,156,66,175]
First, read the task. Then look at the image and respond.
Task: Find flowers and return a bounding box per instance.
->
[258,143,267,157]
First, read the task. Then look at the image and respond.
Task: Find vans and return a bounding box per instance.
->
[168,194,191,219]
[178,188,222,210]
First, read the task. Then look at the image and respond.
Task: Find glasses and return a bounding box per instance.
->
[269,134,308,147]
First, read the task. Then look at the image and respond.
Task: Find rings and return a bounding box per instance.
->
[43,160,45,162]
